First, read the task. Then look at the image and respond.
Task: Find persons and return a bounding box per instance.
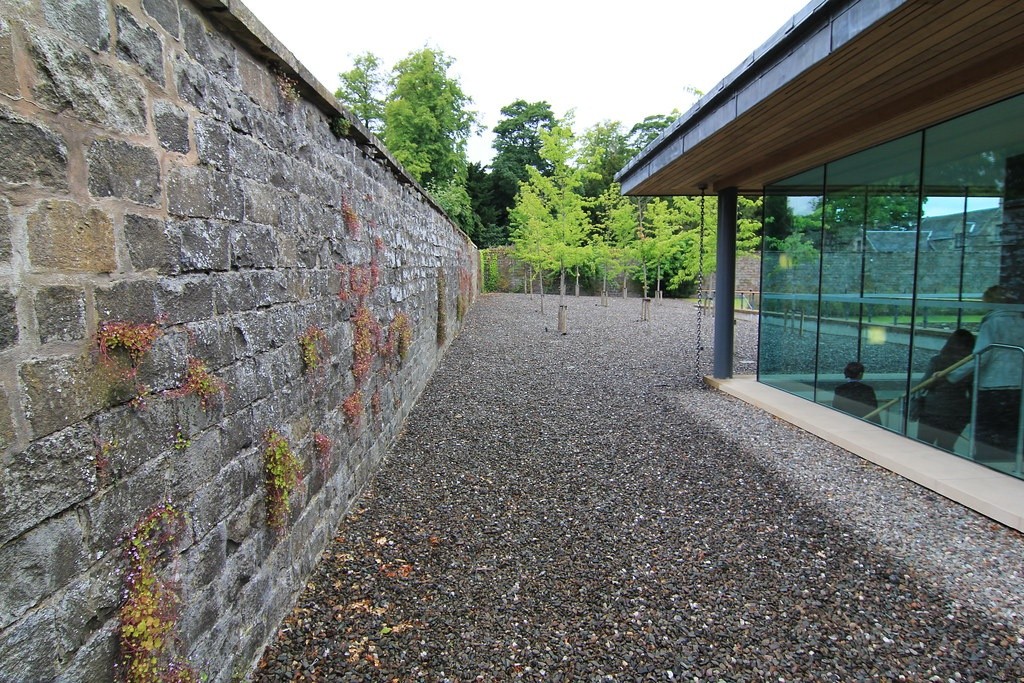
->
[831,361,882,427]
[929,285,1024,464]
[915,327,977,453]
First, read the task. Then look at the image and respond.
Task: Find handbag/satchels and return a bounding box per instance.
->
[909,396,924,421]
